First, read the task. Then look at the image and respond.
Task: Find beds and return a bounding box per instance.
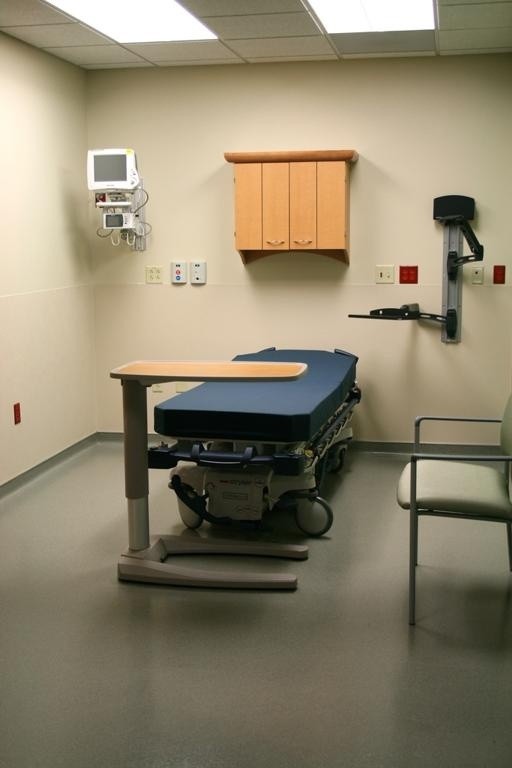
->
[149,349,362,539]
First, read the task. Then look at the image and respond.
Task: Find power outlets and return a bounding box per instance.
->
[190,260,207,284]
[147,266,162,284]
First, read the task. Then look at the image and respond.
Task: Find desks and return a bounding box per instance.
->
[109,361,309,591]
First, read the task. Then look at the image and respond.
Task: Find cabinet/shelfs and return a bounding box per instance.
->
[223,148,359,265]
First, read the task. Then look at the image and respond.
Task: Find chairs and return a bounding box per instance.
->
[398,387,511,625]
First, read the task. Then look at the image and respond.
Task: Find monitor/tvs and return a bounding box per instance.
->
[86,149,140,190]
